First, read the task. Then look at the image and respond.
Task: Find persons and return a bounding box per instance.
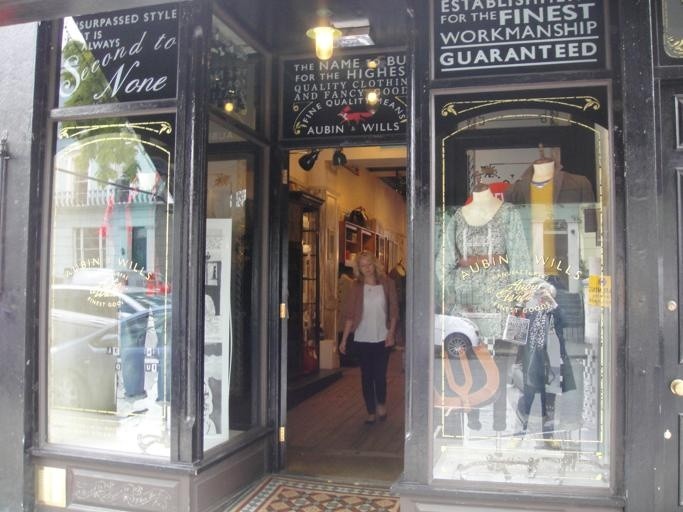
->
[501,156,596,450]
[337,249,400,424]
[436,184,533,449]
[508,278,578,449]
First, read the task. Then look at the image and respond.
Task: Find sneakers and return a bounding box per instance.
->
[545,439,560,448]
[365,411,387,424]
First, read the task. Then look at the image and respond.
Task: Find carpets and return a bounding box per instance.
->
[223,473,401,512]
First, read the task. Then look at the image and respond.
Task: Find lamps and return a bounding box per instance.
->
[305,1,344,61]
[297,148,348,171]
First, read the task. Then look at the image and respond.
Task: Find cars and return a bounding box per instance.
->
[433,312,482,363]
[48,267,168,350]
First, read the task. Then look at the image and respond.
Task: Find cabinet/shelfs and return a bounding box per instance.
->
[289,190,326,376]
[339,219,401,366]
